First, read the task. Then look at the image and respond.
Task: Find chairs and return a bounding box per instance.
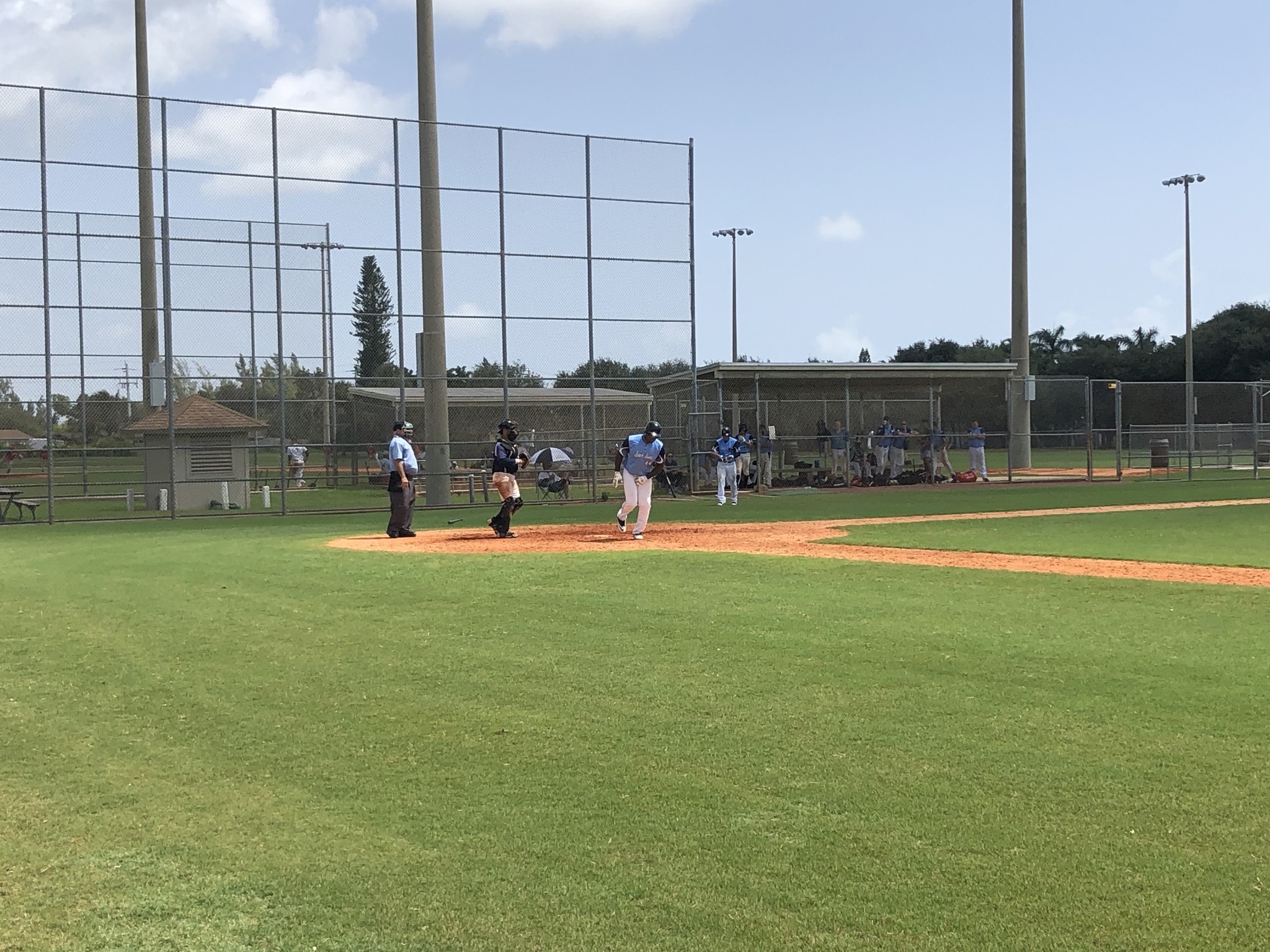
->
[538,472,564,500]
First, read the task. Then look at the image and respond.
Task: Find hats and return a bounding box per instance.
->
[884,416,889,421]
[741,424,747,428]
[393,421,404,431]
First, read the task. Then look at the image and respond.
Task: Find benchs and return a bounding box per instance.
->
[772,463,944,473]
[12,500,40,520]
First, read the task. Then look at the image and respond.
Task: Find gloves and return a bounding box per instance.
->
[634,475,648,486]
[613,471,623,488]
[738,435,744,442]
[720,455,732,464]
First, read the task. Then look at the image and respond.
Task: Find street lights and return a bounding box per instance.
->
[1162,172,1206,449]
[711,227,755,438]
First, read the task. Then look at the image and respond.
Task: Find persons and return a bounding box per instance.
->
[382,447,492,475]
[538,462,572,499]
[286,437,309,488]
[655,424,774,506]
[966,420,990,482]
[831,415,955,483]
[486,419,531,539]
[613,420,664,540]
[386,420,418,539]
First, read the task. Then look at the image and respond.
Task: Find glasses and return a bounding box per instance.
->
[901,423,907,425]
[668,455,673,458]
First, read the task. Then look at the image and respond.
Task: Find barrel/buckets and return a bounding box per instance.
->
[1149,437,1169,467]
[782,441,798,465]
[1257,438,1270,462]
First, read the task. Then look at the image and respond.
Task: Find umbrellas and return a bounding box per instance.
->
[528,447,577,464]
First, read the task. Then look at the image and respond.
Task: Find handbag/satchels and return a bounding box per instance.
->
[387,479,402,492]
[773,468,977,488]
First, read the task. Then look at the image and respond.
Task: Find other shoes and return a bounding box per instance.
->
[983,478,989,482]
[401,532,416,537]
[717,502,736,506]
[389,534,398,538]
[950,479,955,483]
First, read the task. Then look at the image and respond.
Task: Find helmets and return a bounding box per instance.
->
[722,426,730,436]
[644,421,663,437]
[497,421,515,433]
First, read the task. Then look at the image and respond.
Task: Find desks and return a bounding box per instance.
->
[0,488,23,522]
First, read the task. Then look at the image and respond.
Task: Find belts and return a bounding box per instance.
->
[719,460,731,463]
[393,472,413,478]
[741,451,750,454]
[879,446,887,448]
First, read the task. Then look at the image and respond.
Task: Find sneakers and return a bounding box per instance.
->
[487,517,498,534]
[617,517,643,539]
[497,532,514,537]
